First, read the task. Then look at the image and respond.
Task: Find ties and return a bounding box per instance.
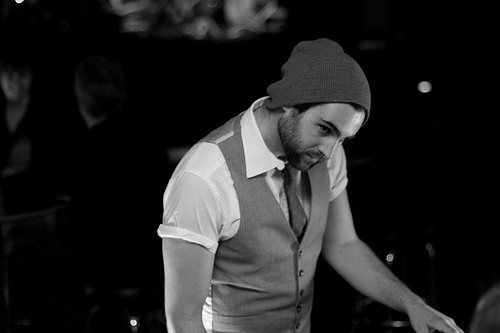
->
[280,162,309,244]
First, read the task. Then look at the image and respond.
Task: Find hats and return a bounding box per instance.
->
[265,38,371,128]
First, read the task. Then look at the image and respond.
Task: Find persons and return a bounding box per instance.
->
[156,38,466,332]
[1,41,143,331]
